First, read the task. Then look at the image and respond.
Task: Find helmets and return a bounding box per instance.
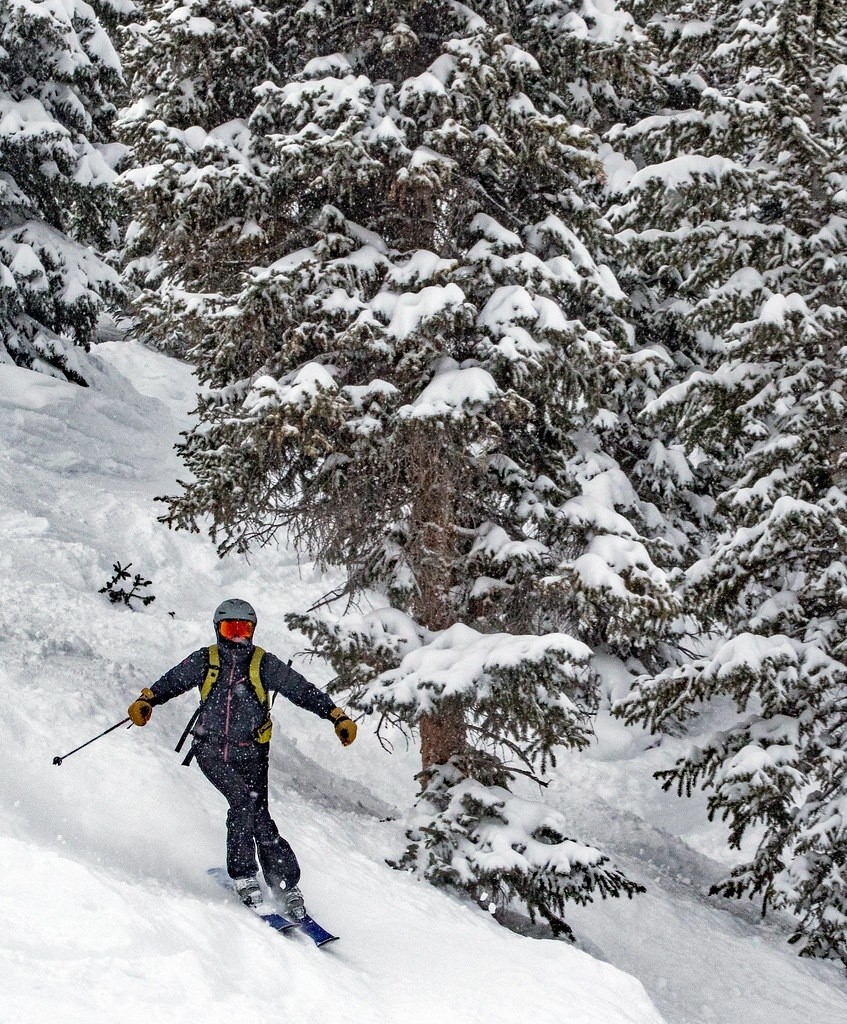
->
[213,599,257,631]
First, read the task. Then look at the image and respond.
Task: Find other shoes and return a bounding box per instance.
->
[258,821,300,879]
[225,810,258,879]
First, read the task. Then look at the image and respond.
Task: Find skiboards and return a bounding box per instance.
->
[208,867,342,949]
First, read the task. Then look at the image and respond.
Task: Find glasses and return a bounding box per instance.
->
[218,619,253,640]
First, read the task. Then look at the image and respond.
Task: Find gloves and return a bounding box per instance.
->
[128,688,154,726]
[328,707,357,746]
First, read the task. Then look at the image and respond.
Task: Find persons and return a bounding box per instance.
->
[128,599,358,917]
